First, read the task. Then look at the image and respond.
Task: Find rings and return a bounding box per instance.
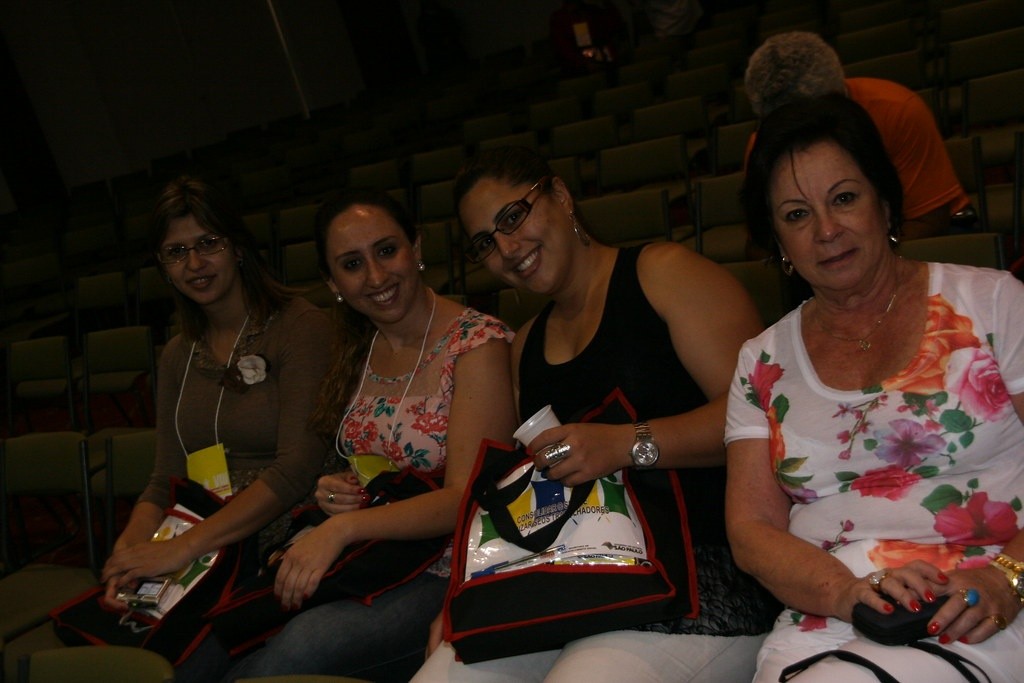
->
[544,441,571,465]
[958,584,979,606]
[991,614,1006,629]
[869,570,888,590]
[328,492,335,504]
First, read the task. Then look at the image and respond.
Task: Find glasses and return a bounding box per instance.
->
[159,232,228,263]
[464,175,546,264]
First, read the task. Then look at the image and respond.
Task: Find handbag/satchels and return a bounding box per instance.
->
[779,584,992,682]
[444,388,698,667]
[204,464,453,661]
[49,473,261,670]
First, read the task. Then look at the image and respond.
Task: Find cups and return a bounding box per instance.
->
[513,404,562,449]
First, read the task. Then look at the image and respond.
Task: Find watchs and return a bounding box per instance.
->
[990,552,1024,601]
[628,422,659,470]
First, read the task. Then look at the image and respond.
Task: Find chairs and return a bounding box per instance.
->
[0,0,1024,683]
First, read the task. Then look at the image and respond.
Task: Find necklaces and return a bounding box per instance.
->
[816,256,902,352]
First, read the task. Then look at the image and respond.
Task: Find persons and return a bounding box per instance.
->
[630,0,704,40]
[550,0,618,89]
[724,93,1024,683]
[100,176,339,683]
[170,185,516,683]
[743,31,970,244]
[416,0,470,73]
[408,144,785,683]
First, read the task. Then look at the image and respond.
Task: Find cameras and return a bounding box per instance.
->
[115,576,172,608]
